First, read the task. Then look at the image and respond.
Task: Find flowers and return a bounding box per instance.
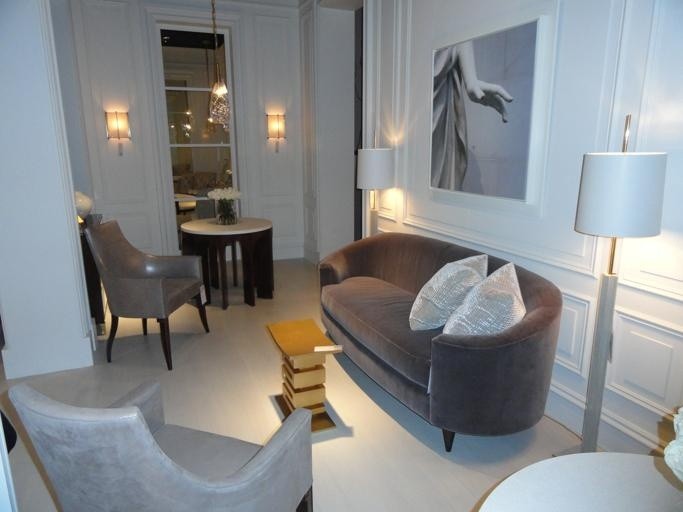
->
[207,187,242,224]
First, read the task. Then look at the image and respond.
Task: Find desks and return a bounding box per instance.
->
[479,454,683,512]
[180,219,273,309]
[175,194,196,234]
[267,317,343,436]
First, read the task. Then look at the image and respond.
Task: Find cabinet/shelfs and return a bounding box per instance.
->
[81,234,107,336]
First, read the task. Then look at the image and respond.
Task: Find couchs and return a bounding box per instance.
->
[320,232,563,453]
[177,169,233,197]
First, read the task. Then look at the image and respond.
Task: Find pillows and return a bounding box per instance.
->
[427,261,526,395]
[408,254,488,332]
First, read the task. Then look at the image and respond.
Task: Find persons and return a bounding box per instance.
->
[433,39,514,192]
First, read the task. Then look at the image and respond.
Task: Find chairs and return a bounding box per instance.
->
[8,382,313,512]
[84,220,210,371]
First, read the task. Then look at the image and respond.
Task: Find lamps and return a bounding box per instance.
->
[209,0,230,125]
[266,114,286,153]
[575,114,668,452]
[357,149,394,237]
[105,111,131,155]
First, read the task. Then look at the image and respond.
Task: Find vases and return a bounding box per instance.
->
[215,200,239,224]
